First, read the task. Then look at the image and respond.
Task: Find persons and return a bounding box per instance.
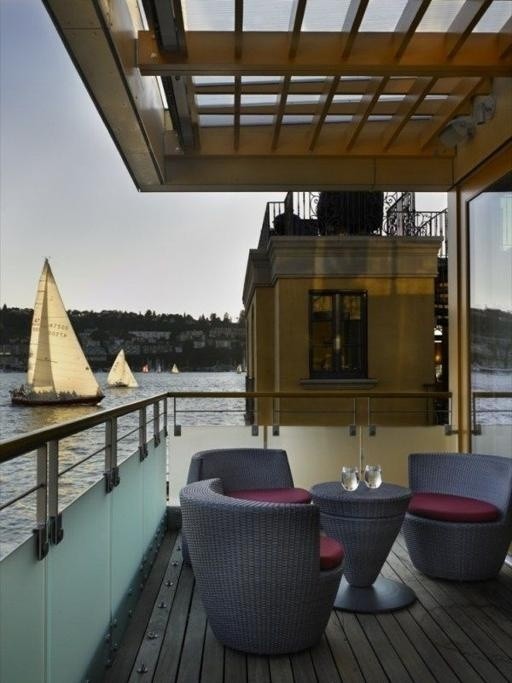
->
[15,389,18,396]
[19,384,26,397]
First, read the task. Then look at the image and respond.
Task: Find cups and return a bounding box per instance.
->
[363,464,383,490]
[341,465,360,491]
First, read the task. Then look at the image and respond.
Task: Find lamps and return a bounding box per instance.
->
[439,118,474,146]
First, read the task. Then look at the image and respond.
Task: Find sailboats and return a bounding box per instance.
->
[141,363,150,373]
[236,364,242,374]
[156,364,162,373]
[170,362,180,374]
[8,255,108,410]
[105,347,143,390]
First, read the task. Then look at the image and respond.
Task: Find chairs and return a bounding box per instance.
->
[180,478,346,654]
[404,453,512,580]
[181,448,313,566]
